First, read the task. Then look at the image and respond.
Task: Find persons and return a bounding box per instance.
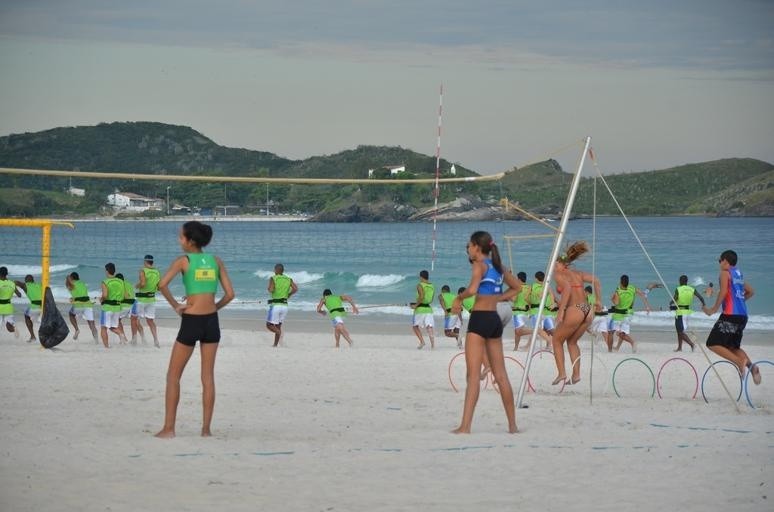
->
[317,289,359,349]
[114,272,147,345]
[97,263,129,348]
[133,254,162,346]
[551,240,603,386]
[0,266,22,333]
[465,242,513,382]
[64,271,102,345]
[438,270,715,353]
[265,263,299,349]
[12,274,44,344]
[701,250,762,385]
[409,269,435,351]
[448,230,525,434]
[152,220,237,437]
